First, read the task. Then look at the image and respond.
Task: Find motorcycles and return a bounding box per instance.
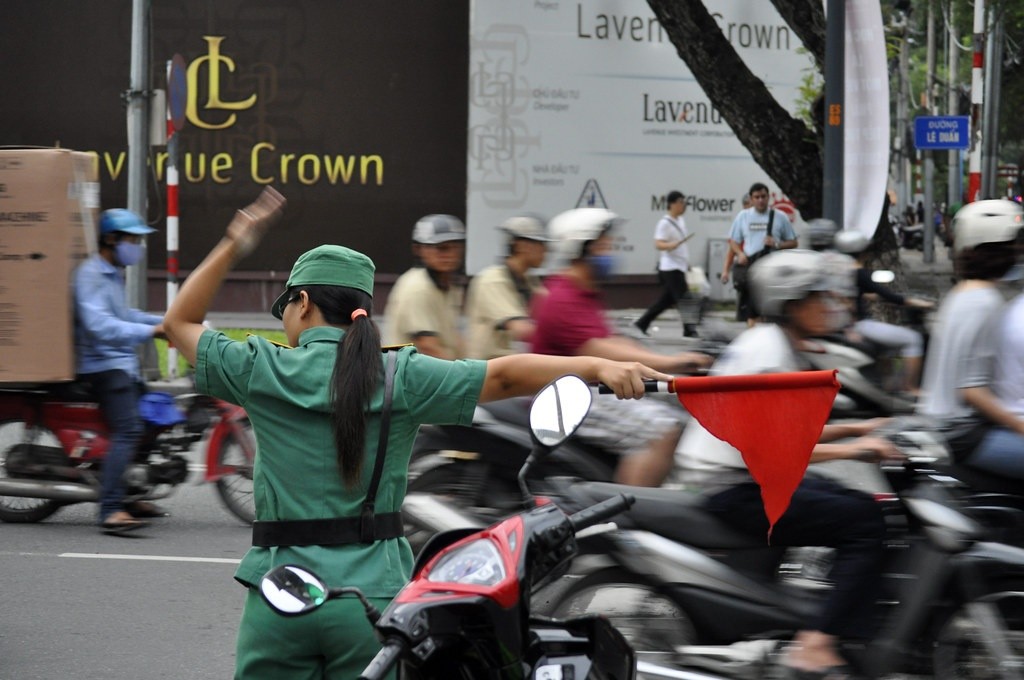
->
[253,371,643,680]
[403,240,1024,680]
[0,325,259,529]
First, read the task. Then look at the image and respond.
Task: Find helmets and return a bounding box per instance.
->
[502,217,558,242]
[746,249,856,317]
[809,219,838,247]
[411,213,465,245]
[549,206,615,260]
[100,208,158,236]
[949,197,1023,256]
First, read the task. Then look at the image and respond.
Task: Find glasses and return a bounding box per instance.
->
[278,289,311,317]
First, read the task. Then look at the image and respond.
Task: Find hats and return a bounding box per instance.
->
[270,244,375,320]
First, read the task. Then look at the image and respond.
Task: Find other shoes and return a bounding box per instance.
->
[759,654,869,680]
[629,320,651,341]
[683,331,704,341]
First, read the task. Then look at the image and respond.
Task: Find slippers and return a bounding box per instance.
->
[131,501,169,517]
[101,512,151,533]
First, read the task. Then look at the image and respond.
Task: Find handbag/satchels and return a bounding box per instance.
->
[731,248,770,297]
[685,267,709,302]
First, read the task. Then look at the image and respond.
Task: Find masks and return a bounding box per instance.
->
[113,240,146,266]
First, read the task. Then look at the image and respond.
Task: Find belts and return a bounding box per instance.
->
[251,512,403,546]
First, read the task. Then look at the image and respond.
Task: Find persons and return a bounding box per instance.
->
[529,208,714,487]
[163,186,674,680]
[381,213,466,362]
[720,184,798,322]
[807,219,932,399]
[462,216,549,428]
[631,192,698,338]
[917,199,1024,483]
[674,247,905,680]
[72,208,168,530]
[916,201,924,222]
[904,201,915,224]
